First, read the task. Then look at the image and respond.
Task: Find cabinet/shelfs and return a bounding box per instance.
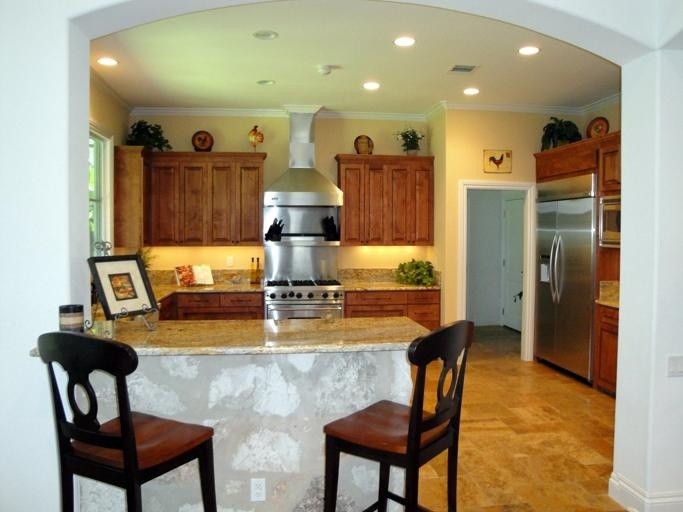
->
[149,151,207,247]
[205,152,266,244]
[592,301,618,396]
[531,137,596,182]
[384,157,434,245]
[598,136,621,195]
[176,283,265,321]
[344,280,440,332]
[335,154,384,247]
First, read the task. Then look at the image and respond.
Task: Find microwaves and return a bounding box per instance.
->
[598,196,620,246]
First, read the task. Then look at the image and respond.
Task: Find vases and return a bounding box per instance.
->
[403,149,419,156]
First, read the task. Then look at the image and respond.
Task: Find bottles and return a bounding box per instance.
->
[249,257,261,285]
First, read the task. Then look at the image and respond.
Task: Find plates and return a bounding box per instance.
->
[190,130,214,152]
[354,135,374,155]
[585,116,609,139]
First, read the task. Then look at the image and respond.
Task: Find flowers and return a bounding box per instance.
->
[393,124,426,150]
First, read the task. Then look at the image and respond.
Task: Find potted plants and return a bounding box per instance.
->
[128,119,173,165]
[539,115,582,151]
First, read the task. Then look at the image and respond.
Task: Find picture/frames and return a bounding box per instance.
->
[86,254,159,321]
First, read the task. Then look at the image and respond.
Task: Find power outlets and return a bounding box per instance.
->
[249,477,266,503]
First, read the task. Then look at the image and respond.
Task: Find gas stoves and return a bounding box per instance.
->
[263,280,345,301]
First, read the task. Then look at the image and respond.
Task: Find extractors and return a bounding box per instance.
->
[262,112,344,209]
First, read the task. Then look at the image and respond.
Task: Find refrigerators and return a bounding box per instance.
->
[533,196,595,385]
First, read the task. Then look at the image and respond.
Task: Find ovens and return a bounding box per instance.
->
[264,301,344,320]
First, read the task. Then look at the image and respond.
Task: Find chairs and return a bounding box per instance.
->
[323,320,477,511]
[36,332,218,512]
